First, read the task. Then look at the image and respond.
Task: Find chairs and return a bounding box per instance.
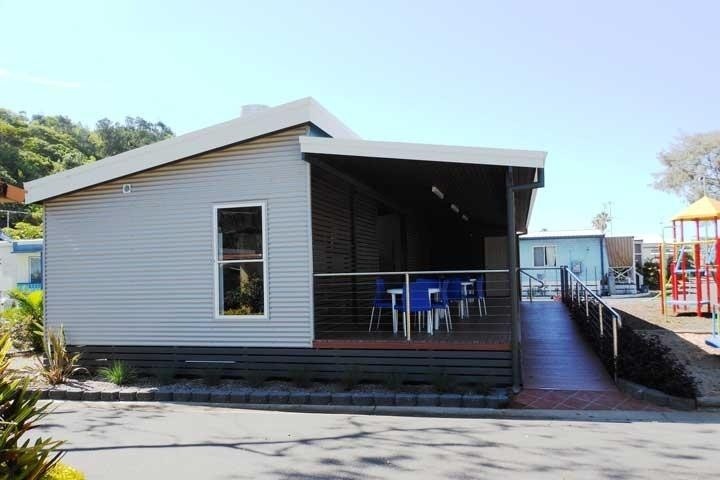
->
[367,274,487,338]
